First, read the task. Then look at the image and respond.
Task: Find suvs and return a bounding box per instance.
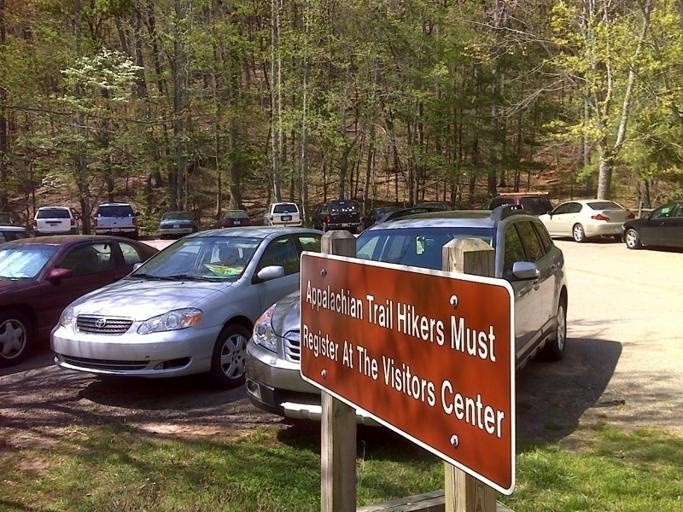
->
[94,201,139,239]
[262,202,304,228]
[32,205,80,236]
[216,209,250,228]
[312,201,365,233]
[244,204,567,443]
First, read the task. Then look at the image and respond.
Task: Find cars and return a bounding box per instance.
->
[159,211,198,238]
[621,200,682,255]
[537,200,634,243]
[1,235,159,367]
[49,227,326,389]
[365,206,400,229]
[1,213,29,242]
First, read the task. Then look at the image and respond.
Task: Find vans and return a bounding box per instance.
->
[475,194,553,216]
